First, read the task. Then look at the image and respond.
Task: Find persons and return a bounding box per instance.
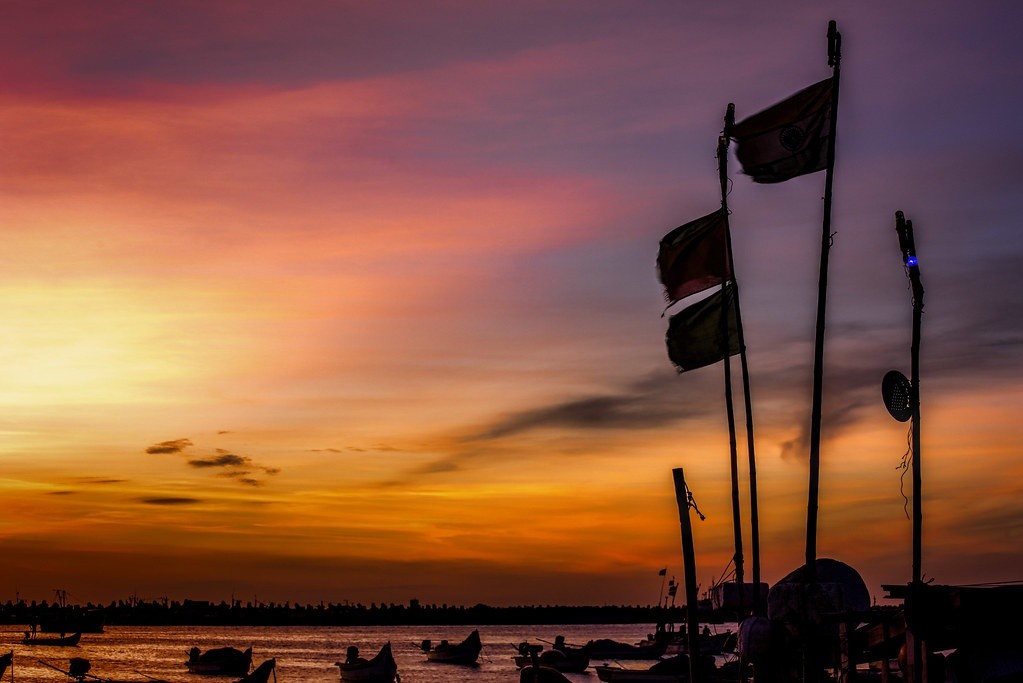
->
[30,621,37,639]
[703,625,711,636]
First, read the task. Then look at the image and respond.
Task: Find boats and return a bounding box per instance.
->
[22,629,82,646]
[183,646,252,675]
[333,640,401,683]
[39,589,108,633]
[511,639,594,672]
[519,663,573,683]
[583,582,741,683]
[232,657,277,683]
[0,649,14,680]
[421,628,483,663]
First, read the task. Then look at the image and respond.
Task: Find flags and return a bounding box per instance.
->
[655,208,731,318]
[666,283,747,375]
[731,76,839,184]
